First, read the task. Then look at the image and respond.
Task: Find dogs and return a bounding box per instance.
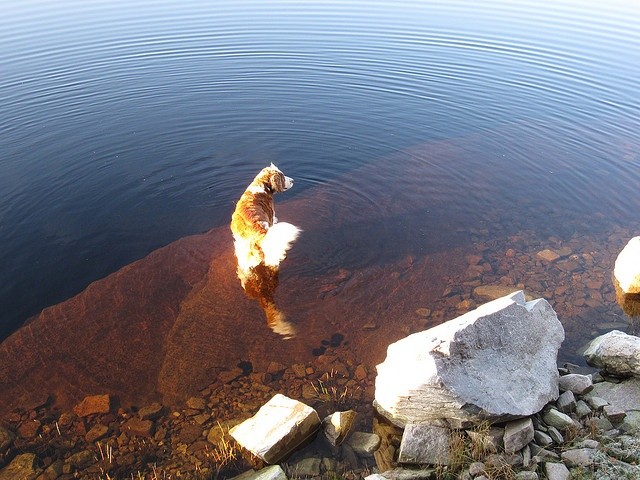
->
[230,160,303,282]
